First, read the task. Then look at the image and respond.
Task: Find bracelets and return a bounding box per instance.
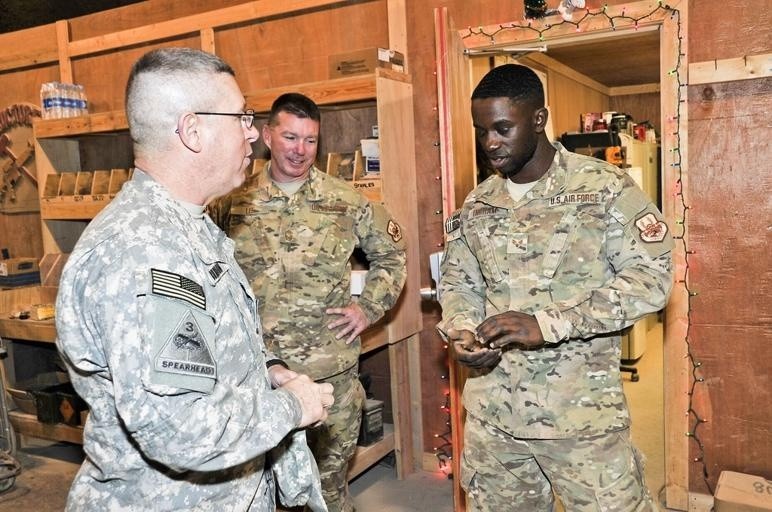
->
[266,359,289,369]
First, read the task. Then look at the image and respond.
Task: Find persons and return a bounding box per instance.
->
[51,45,337,512]
[201,90,410,512]
[434,63,676,511]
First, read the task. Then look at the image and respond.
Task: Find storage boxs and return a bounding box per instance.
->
[327,47,404,76]
[712,470,771,512]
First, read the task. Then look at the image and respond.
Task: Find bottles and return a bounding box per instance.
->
[41,81,89,119]
[592,113,627,133]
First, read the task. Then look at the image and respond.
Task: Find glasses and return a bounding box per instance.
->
[174,108,255,134]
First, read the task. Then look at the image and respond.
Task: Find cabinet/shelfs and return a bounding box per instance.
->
[0,66,423,499]
[564,131,664,380]
